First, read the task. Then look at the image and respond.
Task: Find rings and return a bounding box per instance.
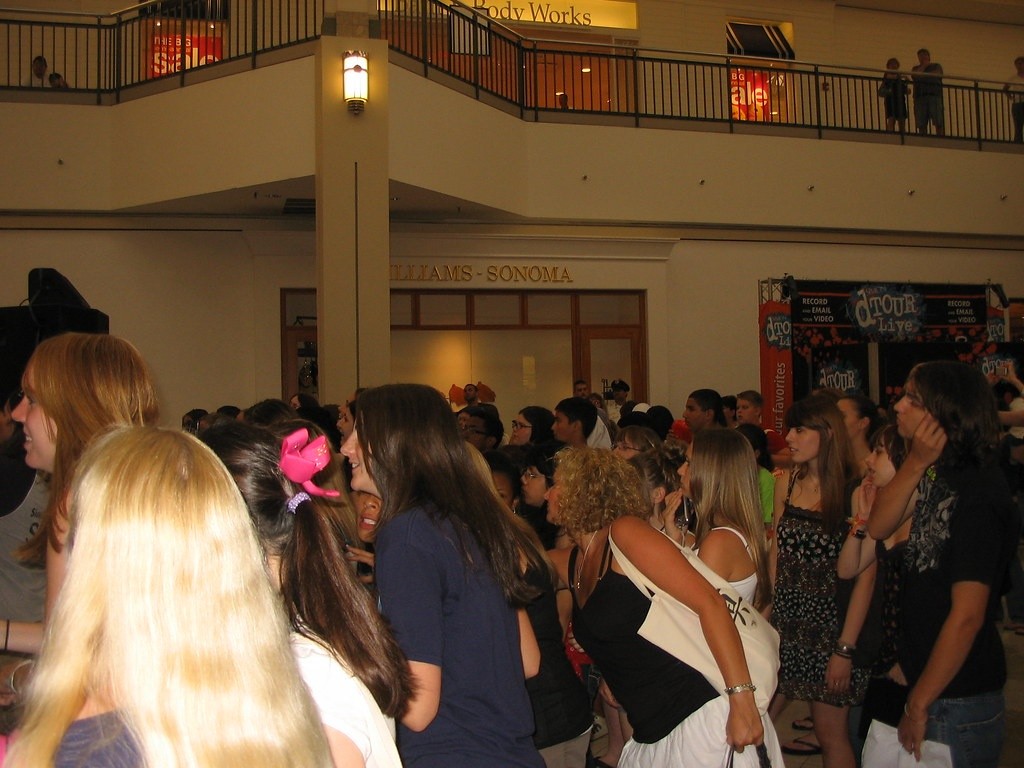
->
[835,683,840,686]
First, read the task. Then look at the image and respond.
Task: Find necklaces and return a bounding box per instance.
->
[577,531,597,589]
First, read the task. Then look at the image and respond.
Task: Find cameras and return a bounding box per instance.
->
[675,497,691,528]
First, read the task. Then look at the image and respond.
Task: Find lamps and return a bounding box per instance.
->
[342,49,369,116]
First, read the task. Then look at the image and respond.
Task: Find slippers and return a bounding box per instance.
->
[781,737,822,754]
[792,717,814,730]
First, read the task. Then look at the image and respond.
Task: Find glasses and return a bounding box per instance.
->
[610,443,644,452]
[521,468,552,480]
[463,426,489,437]
[511,420,532,430]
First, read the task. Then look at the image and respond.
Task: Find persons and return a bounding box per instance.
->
[1003,57,1024,144]
[544,360,1024,768]
[183,393,358,448]
[341,383,593,768]
[911,49,944,135]
[0,333,335,768]
[23,56,64,87]
[194,422,411,768]
[879,58,912,131]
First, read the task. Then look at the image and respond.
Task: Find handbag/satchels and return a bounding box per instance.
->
[861,719,953,768]
[609,521,781,716]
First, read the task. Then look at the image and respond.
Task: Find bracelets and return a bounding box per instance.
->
[905,703,927,724]
[848,515,867,535]
[850,528,864,539]
[724,683,756,695]
[833,639,856,659]
[5,620,10,651]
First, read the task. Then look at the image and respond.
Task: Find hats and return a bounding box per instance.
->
[611,379,629,392]
